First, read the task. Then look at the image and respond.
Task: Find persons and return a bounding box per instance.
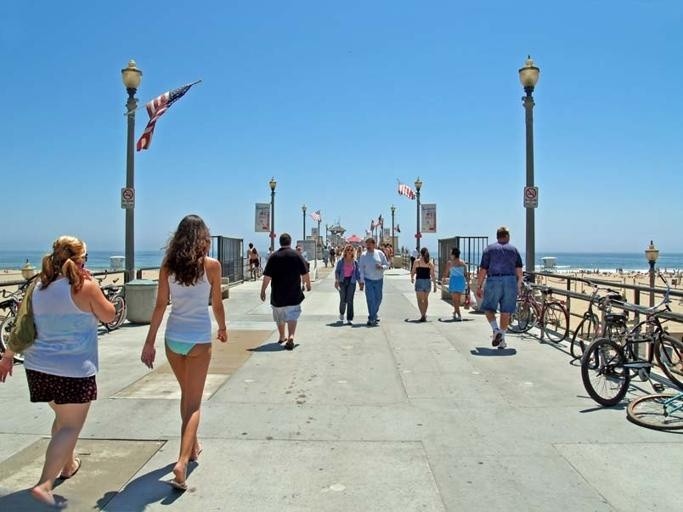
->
[245,242,394,293]
[259,233,313,351]
[475,225,525,348]
[333,244,364,324]
[440,246,470,319]
[140,214,228,495]
[0,231,117,508]
[360,238,389,328]
[410,248,437,322]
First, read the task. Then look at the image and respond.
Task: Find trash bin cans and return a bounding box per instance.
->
[393,256,402,268]
[126,280,158,324]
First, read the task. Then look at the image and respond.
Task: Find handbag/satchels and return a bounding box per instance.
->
[9,282,34,351]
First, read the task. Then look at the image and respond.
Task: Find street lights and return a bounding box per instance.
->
[380,215,383,240]
[390,204,394,254]
[268,177,274,249]
[300,202,306,240]
[413,176,422,254]
[120,57,137,279]
[316,209,320,242]
[642,240,659,337]
[518,54,539,280]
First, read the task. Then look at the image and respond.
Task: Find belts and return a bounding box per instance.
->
[487,273,514,276]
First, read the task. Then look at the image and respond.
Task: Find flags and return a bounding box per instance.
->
[132,82,193,155]
[397,181,415,201]
[309,209,322,223]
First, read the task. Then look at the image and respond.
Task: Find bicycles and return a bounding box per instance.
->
[1,271,126,362]
[502,272,566,346]
[565,268,682,429]
[322,256,334,267]
[250,262,263,282]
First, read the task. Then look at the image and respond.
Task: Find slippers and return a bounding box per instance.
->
[189,448,202,461]
[167,480,188,490]
[286,343,294,347]
[52,495,68,508]
[60,458,80,478]
[278,339,287,342]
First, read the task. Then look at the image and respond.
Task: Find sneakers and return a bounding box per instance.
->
[498,343,507,348]
[340,314,344,320]
[376,314,380,320]
[492,329,502,346]
[348,320,353,324]
[367,320,376,326]
